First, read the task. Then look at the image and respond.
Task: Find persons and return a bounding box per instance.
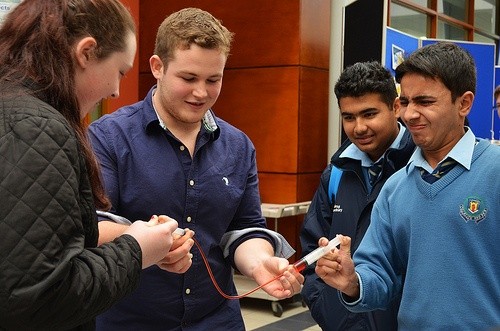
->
[314,41,500,331]
[85,5,306,331]
[493,82,500,120]
[0,1,179,331]
[301,61,417,331]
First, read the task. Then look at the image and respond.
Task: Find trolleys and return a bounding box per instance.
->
[233,202,312,317]
